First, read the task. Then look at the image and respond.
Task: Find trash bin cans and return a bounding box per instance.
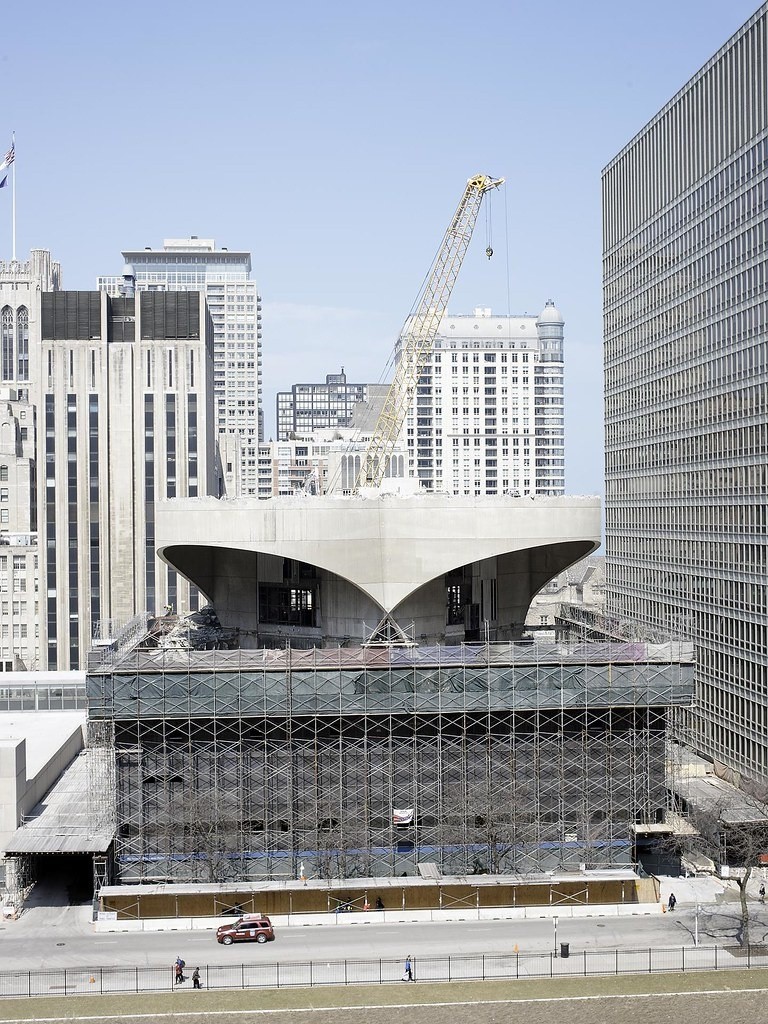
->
[560,942,569,958]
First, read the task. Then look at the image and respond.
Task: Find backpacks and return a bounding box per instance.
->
[180,960,185,968]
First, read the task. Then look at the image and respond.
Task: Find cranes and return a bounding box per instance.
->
[306,169,505,494]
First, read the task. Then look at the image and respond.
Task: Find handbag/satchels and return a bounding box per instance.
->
[402,971,410,980]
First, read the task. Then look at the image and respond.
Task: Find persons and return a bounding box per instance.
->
[193,967,202,988]
[402,955,415,980]
[759,882,766,903]
[375,897,384,909]
[336,897,353,910]
[668,893,677,912]
[234,901,240,913]
[174,957,186,985]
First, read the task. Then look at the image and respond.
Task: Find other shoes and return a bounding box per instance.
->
[174,983,178,985]
[179,979,185,984]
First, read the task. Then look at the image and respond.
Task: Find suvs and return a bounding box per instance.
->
[216,913,274,945]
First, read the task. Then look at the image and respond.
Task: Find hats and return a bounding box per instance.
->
[196,967,200,970]
